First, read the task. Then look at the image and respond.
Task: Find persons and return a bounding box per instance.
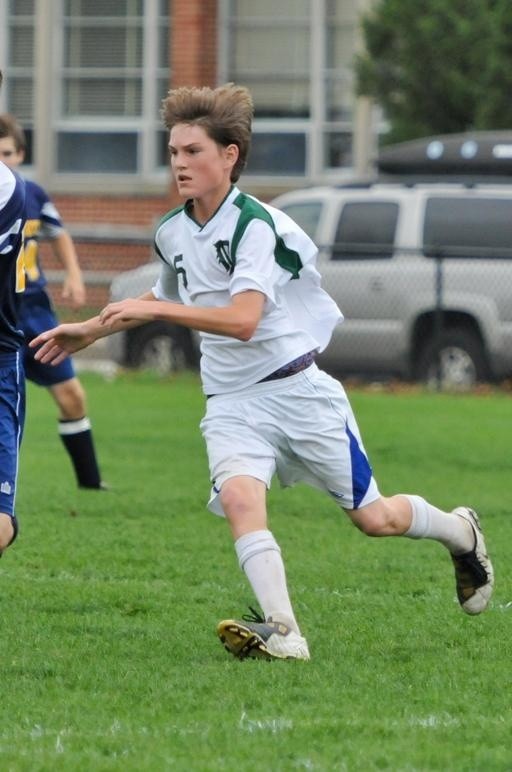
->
[26,82,495,662]
[0,159,27,558]
[1,111,109,492]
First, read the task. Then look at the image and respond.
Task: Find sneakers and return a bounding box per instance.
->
[216,608,312,662]
[448,507,494,617]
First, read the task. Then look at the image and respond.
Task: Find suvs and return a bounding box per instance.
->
[105,126,512,394]
